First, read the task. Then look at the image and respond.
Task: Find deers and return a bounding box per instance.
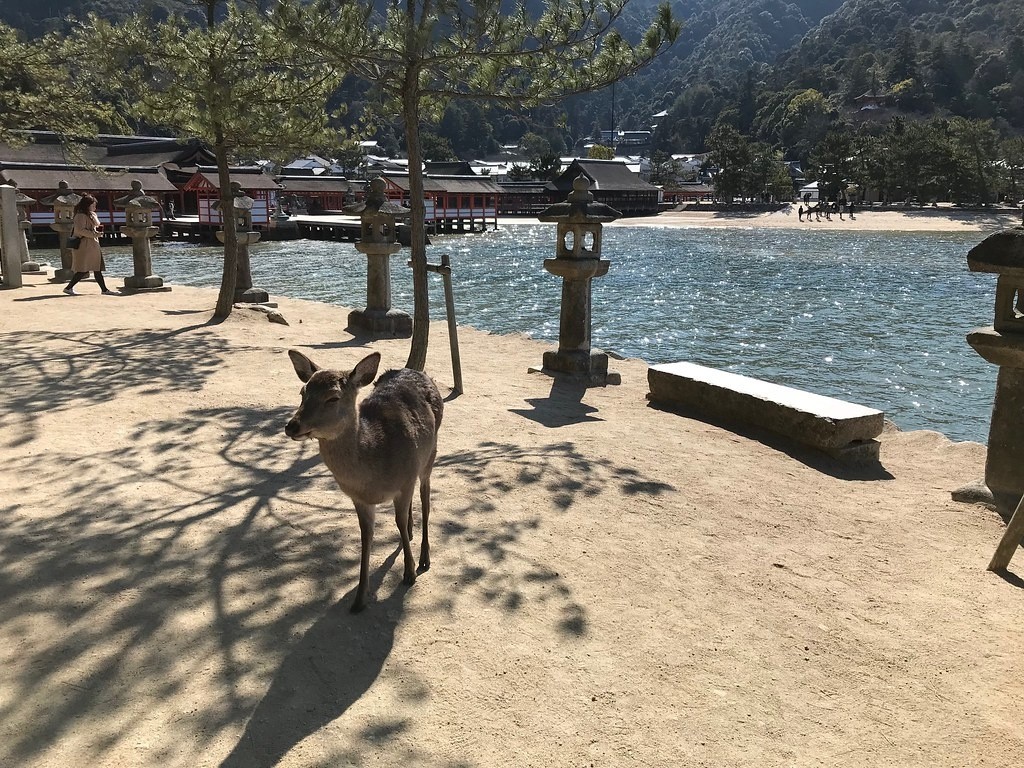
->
[285,348,443,610]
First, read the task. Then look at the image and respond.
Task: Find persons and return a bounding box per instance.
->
[63,195,116,295]
[807,206,812,219]
[816,202,855,219]
[804,194,809,205]
[799,206,803,220]
[167,201,176,220]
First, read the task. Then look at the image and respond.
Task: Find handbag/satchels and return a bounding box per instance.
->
[66,237,80,249]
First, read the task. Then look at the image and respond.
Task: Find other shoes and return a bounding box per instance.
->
[63,288,77,295]
[101,289,115,295]
